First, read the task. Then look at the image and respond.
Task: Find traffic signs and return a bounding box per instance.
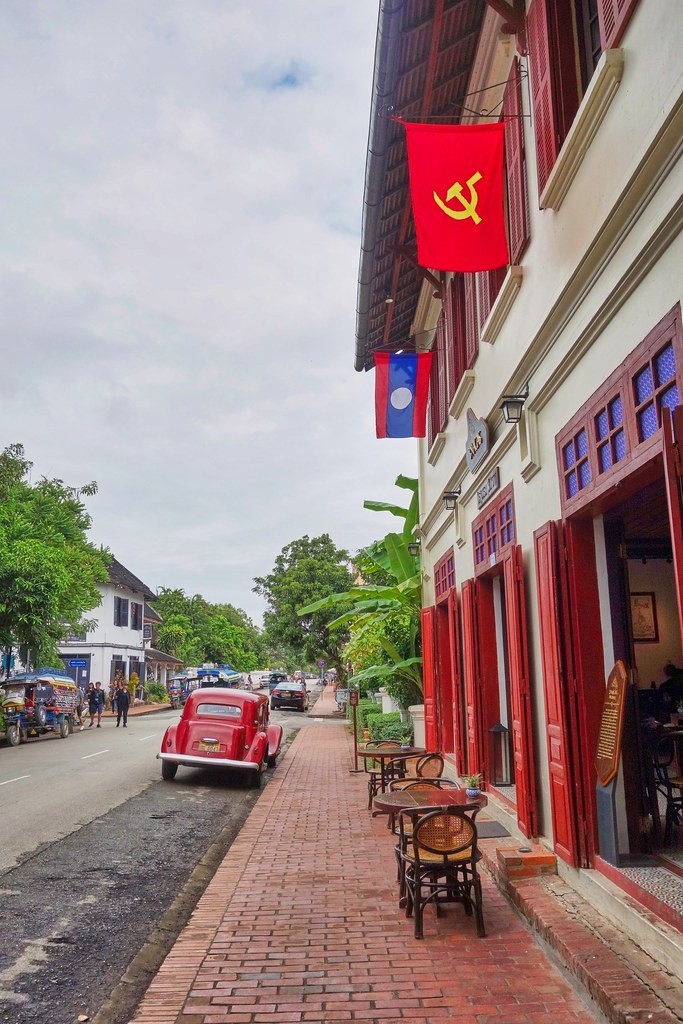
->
[68,659,86,668]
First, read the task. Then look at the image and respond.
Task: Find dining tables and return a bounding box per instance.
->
[373,788,489,904]
[355,747,428,795]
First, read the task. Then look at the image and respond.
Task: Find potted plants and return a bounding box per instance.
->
[398,736,412,751]
[462,772,488,799]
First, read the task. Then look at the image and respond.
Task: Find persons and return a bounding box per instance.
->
[74,688,84,731]
[116,682,131,727]
[108,680,122,715]
[657,662,683,700]
[88,681,106,728]
[85,682,96,717]
[248,675,253,684]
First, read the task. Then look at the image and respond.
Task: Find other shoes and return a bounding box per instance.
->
[116,724,119,727]
[97,724,100,728]
[123,725,127,727]
[89,722,94,727]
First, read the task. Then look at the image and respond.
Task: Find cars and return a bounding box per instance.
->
[269,676,289,695]
[271,682,312,713]
[270,667,339,685]
[155,687,283,791]
[258,674,270,688]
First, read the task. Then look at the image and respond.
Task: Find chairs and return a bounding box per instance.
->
[363,740,409,811]
[384,751,445,835]
[650,730,683,850]
[397,804,488,939]
[387,777,467,885]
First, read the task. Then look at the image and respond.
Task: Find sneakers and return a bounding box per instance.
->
[79,725,84,731]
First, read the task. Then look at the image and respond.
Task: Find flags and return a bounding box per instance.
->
[390,114,510,273]
[370,351,435,439]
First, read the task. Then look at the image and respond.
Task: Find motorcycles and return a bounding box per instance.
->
[2,666,79,747]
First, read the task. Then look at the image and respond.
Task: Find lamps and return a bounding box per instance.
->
[499,398,525,424]
[442,493,458,511]
[407,544,419,556]
